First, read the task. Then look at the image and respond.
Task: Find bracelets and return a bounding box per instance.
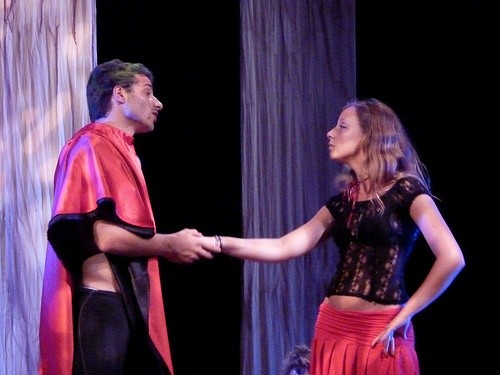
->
[214,235,223,249]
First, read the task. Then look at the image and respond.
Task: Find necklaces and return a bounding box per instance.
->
[357,175,370,184]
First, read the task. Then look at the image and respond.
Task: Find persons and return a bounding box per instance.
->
[197,99,466,375]
[37,60,221,375]
